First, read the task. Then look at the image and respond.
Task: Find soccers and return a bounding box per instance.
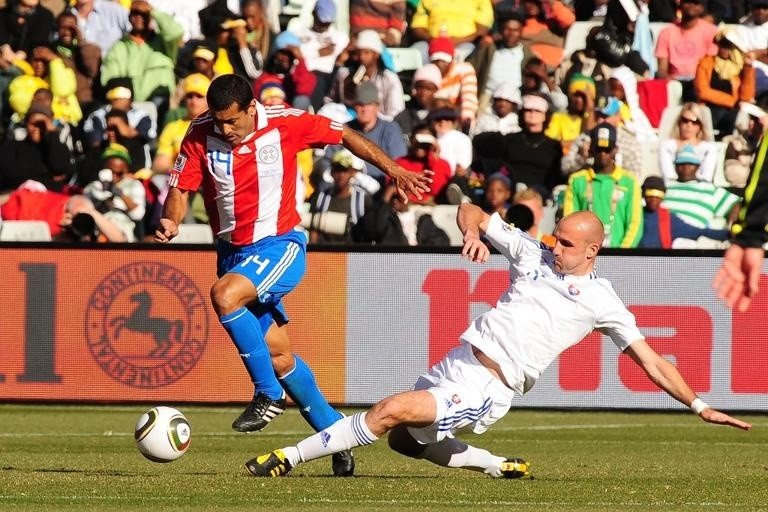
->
[135,406,192,463]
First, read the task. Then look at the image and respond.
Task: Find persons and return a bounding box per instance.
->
[447,91,563,207]
[9,46,84,142]
[250,73,313,198]
[329,28,406,119]
[308,151,372,246]
[214,19,264,85]
[519,56,568,114]
[287,0,349,106]
[194,46,219,80]
[427,39,478,133]
[310,67,393,158]
[562,121,644,249]
[394,61,449,140]
[369,175,449,248]
[638,175,731,249]
[660,145,743,230]
[53,11,101,106]
[514,189,555,248]
[150,73,212,225]
[83,77,158,143]
[56,0,132,65]
[3,103,72,191]
[724,112,768,187]
[560,93,642,180]
[0,43,33,124]
[470,84,521,135]
[712,127,768,313]
[244,202,751,480]
[429,106,473,173]
[693,29,755,131]
[90,108,151,238]
[270,30,317,110]
[317,83,407,189]
[241,0,275,56]
[517,0,577,75]
[659,102,716,183]
[471,12,532,112]
[334,0,406,68]
[61,143,146,244]
[153,73,436,475]
[741,0,768,96]
[99,0,181,112]
[0,0,54,61]
[31,89,73,152]
[478,162,515,253]
[654,0,717,101]
[609,65,650,137]
[409,0,494,49]
[383,123,449,207]
[593,0,649,82]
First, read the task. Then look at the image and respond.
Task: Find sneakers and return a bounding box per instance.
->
[502,457,529,479]
[232,389,292,433]
[332,448,354,477]
[245,448,290,479]
[447,183,463,204]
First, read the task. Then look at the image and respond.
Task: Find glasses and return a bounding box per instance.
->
[185,92,204,99]
[111,169,124,180]
[679,116,700,126]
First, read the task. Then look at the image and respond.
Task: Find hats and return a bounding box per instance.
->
[432,106,455,124]
[673,144,701,166]
[313,0,337,23]
[256,76,284,99]
[183,73,210,96]
[492,79,521,107]
[412,63,441,89]
[568,74,596,97]
[641,175,666,198]
[330,151,354,170]
[275,32,300,49]
[589,123,617,152]
[65,196,93,217]
[523,95,547,113]
[428,37,454,64]
[100,144,131,161]
[354,82,380,105]
[192,39,217,60]
[282,1,302,15]
[106,81,132,101]
[354,30,384,53]
